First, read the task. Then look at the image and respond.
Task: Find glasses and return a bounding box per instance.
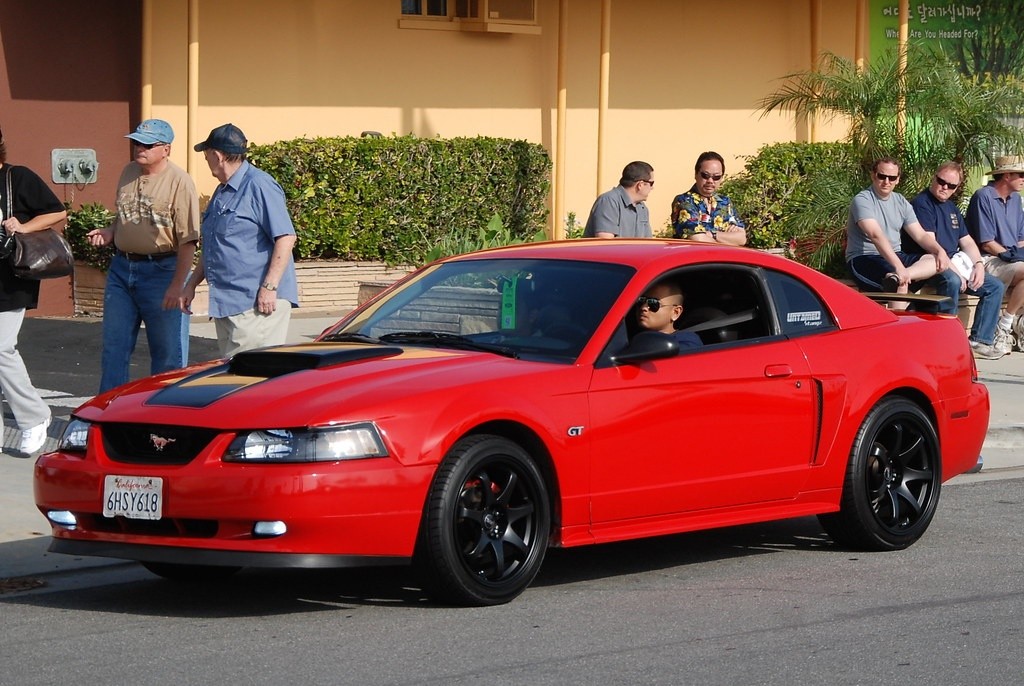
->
[1010,172,1024,178]
[935,174,958,190]
[131,138,167,149]
[636,296,678,313]
[698,169,724,181]
[634,178,655,187]
[874,170,899,182]
[203,148,218,157]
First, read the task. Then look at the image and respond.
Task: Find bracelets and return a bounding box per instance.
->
[973,261,983,267]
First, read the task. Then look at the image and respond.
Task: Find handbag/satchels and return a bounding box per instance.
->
[951,251,985,281]
[5,164,74,280]
[998,244,1024,263]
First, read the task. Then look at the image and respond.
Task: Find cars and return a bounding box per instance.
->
[30,235,991,607]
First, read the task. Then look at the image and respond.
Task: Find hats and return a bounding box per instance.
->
[124,119,175,145]
[194,123,250,154]
[985,156,1024,175]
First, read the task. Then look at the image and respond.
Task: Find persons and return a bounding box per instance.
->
[900,162,1006,360]
[845,157,950,311]
[86,118,200,392]
[635,277,702,351]
[965,155,1024,354]
[671,152,747,248]
[582,161,655,239]
[178,124,299,358]
[0,134,66,456]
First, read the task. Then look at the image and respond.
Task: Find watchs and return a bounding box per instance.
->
[262,281,278,291]
[712,230,718,240]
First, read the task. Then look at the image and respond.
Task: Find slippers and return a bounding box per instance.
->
[879,272,901,294]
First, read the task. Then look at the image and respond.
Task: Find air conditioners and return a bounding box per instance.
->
[465,0,538,25]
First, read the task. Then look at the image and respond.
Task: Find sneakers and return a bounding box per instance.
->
[1014,307,1024,352]
[993,310,1016,355]
[968,339,1005,360]
[20,407,52,458]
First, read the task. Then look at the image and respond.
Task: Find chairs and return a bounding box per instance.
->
[691,308,740,342]
[535,306,593,343]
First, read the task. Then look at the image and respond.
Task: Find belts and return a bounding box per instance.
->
[114,247,177,262]
